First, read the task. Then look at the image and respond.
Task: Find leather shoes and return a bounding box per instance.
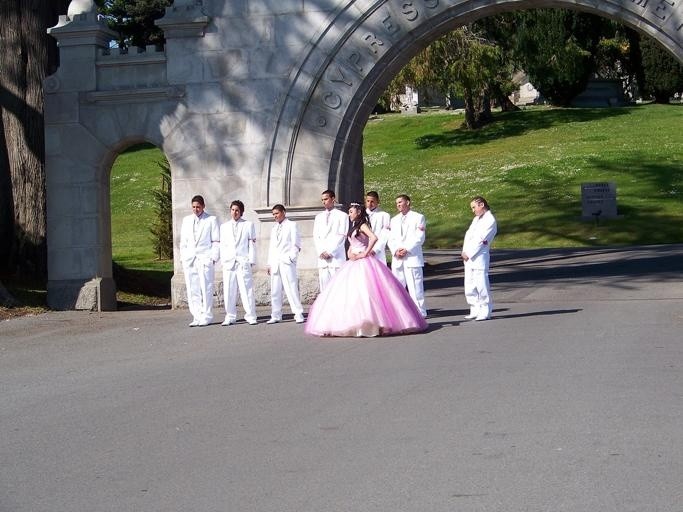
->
[198,319,212,326]
[221,320,235,326]
[249,320,257,325]
[267,319,282,324]
[189,320,199,327]
[475,315,491,320]
[296,317,304,323]
[464,314,477,319]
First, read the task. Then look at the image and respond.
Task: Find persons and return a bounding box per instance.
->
[220,199,257,327]
[385,194,426,319]
[460,195,497,321]
[178,195,219,327]
[312,189,350,294]
[266,204,304,324]
[363,191,390,268]
[304,202,429,339]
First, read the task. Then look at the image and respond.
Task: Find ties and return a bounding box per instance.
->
[401,215,405,237]
[326,213,330,228]
[277,225,282,241]
[233,223,238,241]
[194,218,200,242]
